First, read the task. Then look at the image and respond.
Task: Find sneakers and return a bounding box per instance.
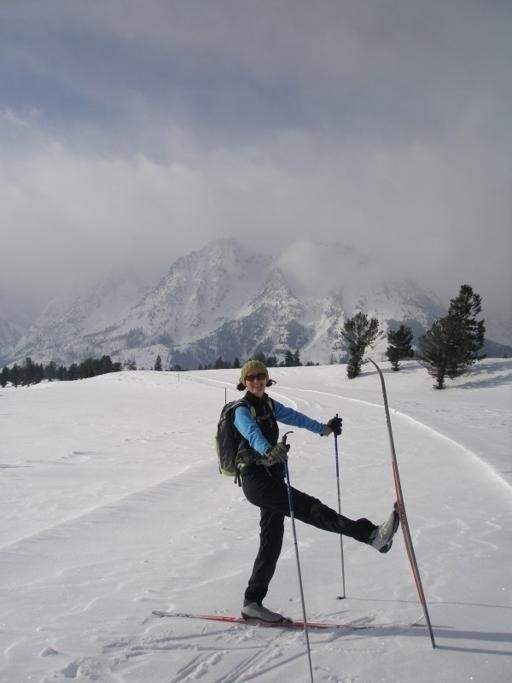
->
[241,598,282,623]
[368,510,399,552]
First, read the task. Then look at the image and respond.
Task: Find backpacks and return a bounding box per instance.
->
[216,397,276,475]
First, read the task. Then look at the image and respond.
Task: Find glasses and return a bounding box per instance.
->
[245,372,266,380]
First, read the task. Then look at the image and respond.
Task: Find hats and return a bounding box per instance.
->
[241,359,269,387]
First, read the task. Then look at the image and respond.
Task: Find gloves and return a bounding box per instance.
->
[263,442,288,464]
[325,416,342,436]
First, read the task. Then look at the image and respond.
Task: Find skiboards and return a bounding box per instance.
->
[152,357,437,650]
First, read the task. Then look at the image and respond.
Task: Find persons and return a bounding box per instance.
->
[231,360,399,623]
